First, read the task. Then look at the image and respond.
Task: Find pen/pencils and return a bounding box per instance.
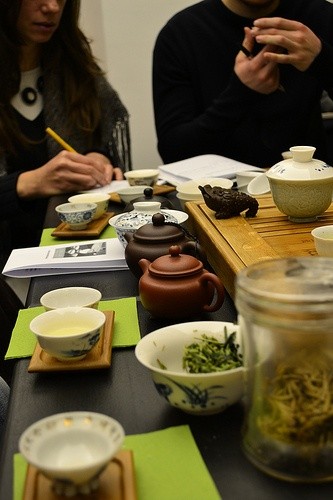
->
[238,44,286,95]
[46,126,77,155]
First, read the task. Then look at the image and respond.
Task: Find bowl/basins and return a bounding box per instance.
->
[116,185,153,204]
[67,193,111,220]
[124,169,160,188]
[176,178,234,212]
[54,202,97,230]
[236,172,271,196]
[135,321,277,416]
[29,307,106,362]
[265,146,333,223]
[39,286,102,311]
[18,412,126,497]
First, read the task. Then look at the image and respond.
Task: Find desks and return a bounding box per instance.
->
[0,189,333,500]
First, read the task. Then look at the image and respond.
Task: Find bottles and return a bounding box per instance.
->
[234,258,333,484]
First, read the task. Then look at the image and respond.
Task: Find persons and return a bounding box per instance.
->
[0,0,132,416]
[150,0,333,168]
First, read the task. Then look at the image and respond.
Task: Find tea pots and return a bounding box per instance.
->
[121,213,208,281]
[137,245,225,321]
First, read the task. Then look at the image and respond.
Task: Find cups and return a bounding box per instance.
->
[108,202,189,248]
[311,226,333,258]
